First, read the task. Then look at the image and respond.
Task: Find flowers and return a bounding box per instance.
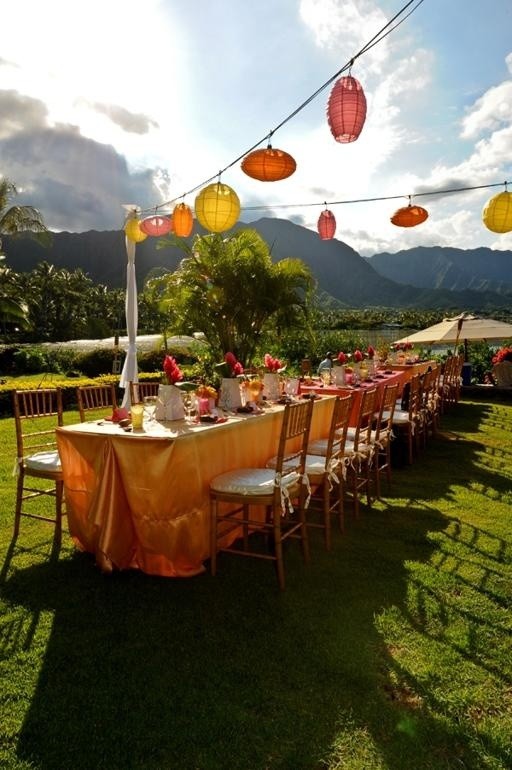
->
[491,347,512,364]
[338,342,415,364]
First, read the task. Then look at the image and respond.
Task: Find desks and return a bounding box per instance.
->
[56,369,405,580]
[360,355,437,384]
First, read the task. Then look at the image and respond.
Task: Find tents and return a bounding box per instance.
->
[140,214,174,238]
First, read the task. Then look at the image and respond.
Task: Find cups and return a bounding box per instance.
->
[323,373,329,387]
[130,404,144,429]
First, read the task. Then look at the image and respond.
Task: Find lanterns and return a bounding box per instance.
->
[124,217,148,244]
[391,205,428,229]
[195,182,241,234]
[327,76,368,142]
[318,209,336,240]
[173,203,194,239]
[240,147,298,182]
[482,192,511,233]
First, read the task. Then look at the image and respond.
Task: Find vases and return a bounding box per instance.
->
[493,361,511,389]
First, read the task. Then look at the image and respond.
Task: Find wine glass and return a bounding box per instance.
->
[143,396,159,424]
[246,375,266,415]
[183,395,197,426]
[281,378,300,405]
[336,358,377,393]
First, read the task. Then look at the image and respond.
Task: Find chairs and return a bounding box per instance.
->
[334,381,399,490]
[266,391,358,552]
[129,378,161,407]
[308,386,379,525]
[301,359,312,376]
[208,396,315,592]
[373,355,464,462]
[12,387,74,564]
[77,382,118,422]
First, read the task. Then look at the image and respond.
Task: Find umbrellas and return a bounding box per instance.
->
[389,312,512,386]
